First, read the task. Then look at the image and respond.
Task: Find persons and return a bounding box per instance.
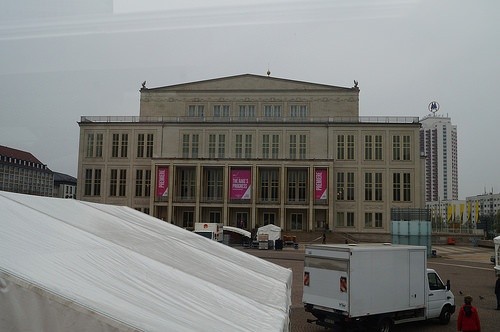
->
[457,296,481,332]
[495,274,500,310]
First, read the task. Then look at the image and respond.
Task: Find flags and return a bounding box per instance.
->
[447,200,481,223]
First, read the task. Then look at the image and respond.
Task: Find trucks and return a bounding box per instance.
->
[193,222,223,244]
[302,242,456,332]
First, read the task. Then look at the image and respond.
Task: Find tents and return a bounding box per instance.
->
[256,224,281,247]
[0,190,293,332]
